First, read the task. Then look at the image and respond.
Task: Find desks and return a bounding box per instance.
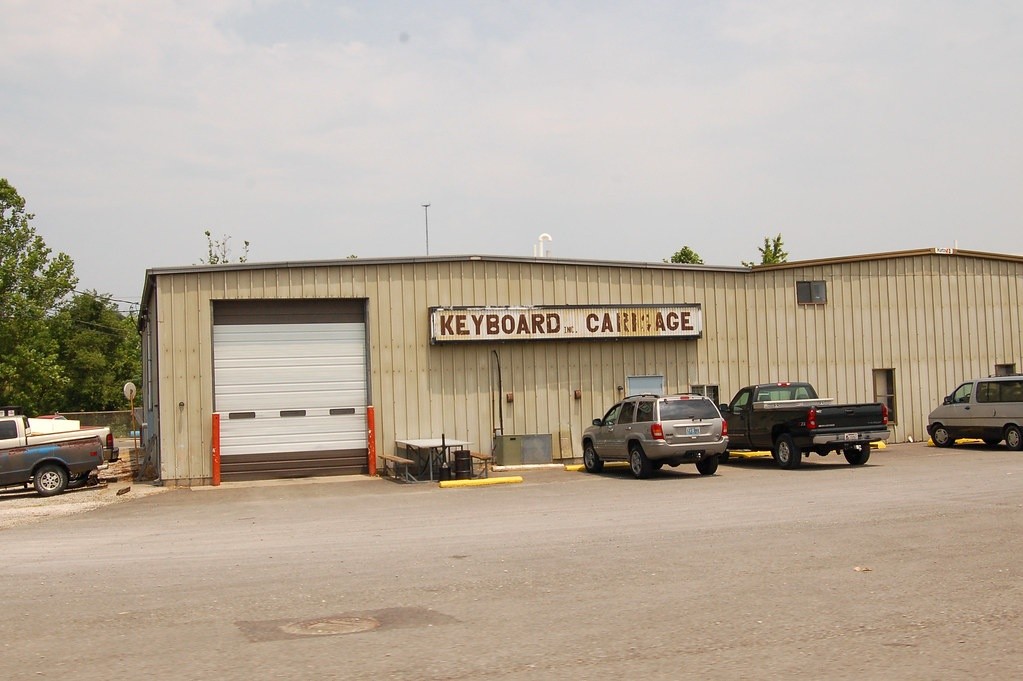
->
[395,439,476,483]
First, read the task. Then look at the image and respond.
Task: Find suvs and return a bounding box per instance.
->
[926,374,1023,451]
[580,394,731,480]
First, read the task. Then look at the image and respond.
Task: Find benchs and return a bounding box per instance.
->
[450,451,496,479]
[377,455,420,484]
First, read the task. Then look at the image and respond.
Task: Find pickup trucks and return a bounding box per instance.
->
[717,382,890,470]
[0,435,106,497]
[0,415,120,464]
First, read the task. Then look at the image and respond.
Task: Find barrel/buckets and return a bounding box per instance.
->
[455,450,471,480]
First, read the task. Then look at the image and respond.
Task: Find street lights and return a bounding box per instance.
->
[422,203,430,255]
